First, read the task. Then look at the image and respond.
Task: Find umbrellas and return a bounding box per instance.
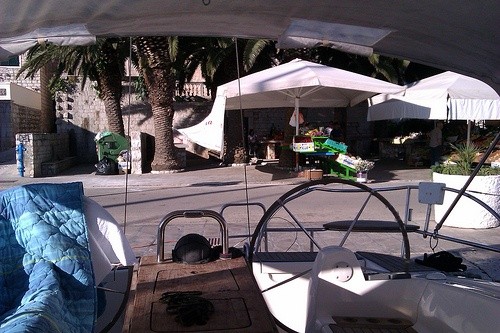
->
[365,69,499,121]
[217,58,405,168]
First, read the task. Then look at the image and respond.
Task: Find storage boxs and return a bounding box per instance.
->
[293,142,314,152]
[335,154,357,167]
[325,139,348,151]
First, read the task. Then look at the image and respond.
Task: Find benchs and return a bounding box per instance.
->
[41,158,73,176]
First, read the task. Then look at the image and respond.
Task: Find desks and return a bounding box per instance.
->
[259,139,281,164]
[304,152,338,178]
[281,149,303,177]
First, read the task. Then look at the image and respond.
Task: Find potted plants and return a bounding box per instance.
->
[430,139,500,229]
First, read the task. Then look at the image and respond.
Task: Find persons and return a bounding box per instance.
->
[426,122,444,169]
[247,129,257,155]
